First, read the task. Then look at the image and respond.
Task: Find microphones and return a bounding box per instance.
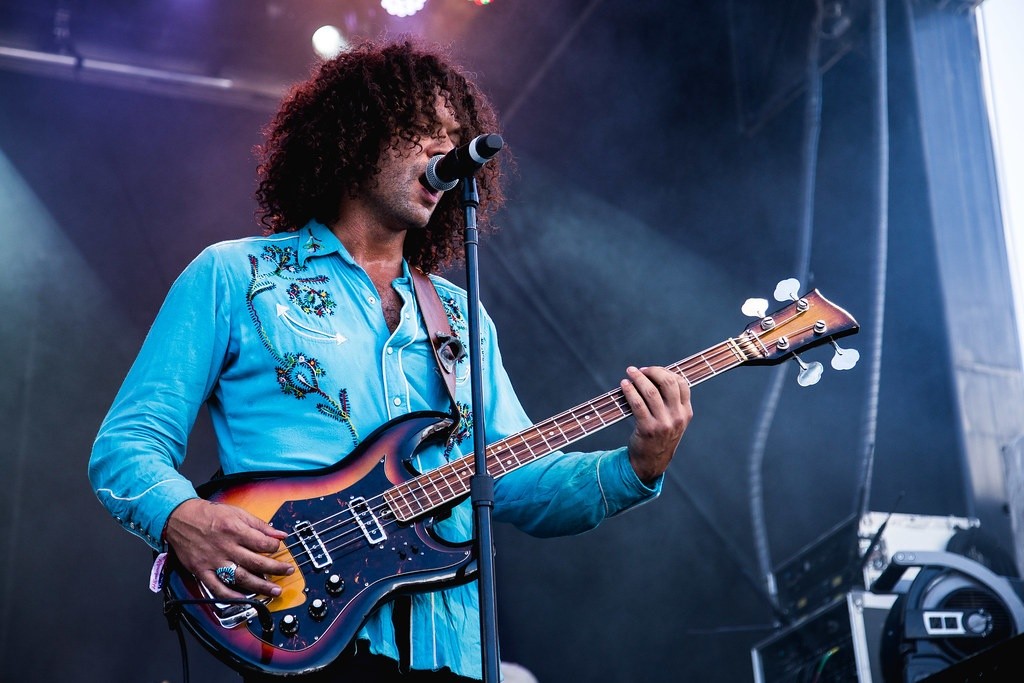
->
[425,133,504,191]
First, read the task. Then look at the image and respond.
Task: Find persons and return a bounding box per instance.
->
[86,25,695,682]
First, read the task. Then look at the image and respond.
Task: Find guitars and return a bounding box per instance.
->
[161,276,863,680]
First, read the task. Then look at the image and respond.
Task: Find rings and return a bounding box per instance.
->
[215,564,236,584]
